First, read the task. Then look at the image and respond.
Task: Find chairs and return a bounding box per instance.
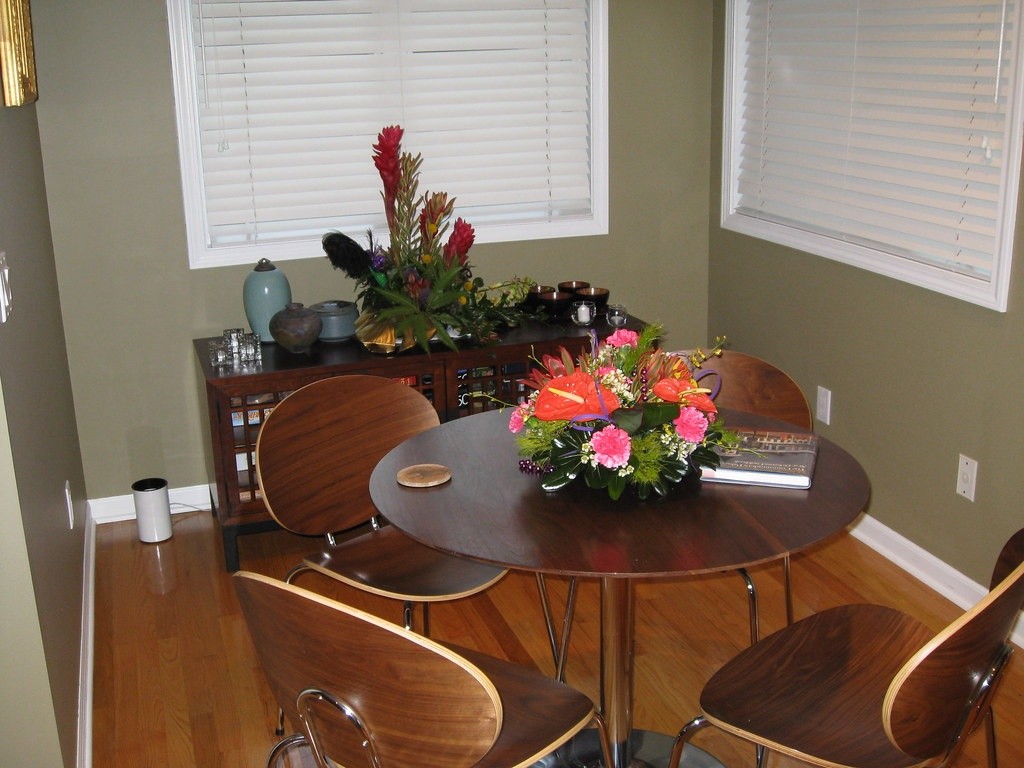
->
[231,569,616,768]
[554,348,814,768]
[254,377,566,685]
[668,529,1024,768]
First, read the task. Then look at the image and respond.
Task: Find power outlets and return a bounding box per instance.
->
[816,385,832,425]
[956,454,979,502]
[64,479,75,529]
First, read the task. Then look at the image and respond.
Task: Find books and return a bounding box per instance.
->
[700,426,820,489]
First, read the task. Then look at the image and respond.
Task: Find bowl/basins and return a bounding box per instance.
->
[575,287,609,309]
[525,286,555,309]
[559,281,590,300]
[540,293,572,314]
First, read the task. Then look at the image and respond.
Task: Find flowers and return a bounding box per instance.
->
[469,317,728,499]
[321,125,537,359]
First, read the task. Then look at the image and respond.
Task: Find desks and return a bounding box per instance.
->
[367,406,872,768]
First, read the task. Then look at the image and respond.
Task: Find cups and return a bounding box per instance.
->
[209,328,262,367]
[572,302,595,325]
[607,304,627,326]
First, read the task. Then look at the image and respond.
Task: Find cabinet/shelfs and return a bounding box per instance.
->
[192,304,659,573]
